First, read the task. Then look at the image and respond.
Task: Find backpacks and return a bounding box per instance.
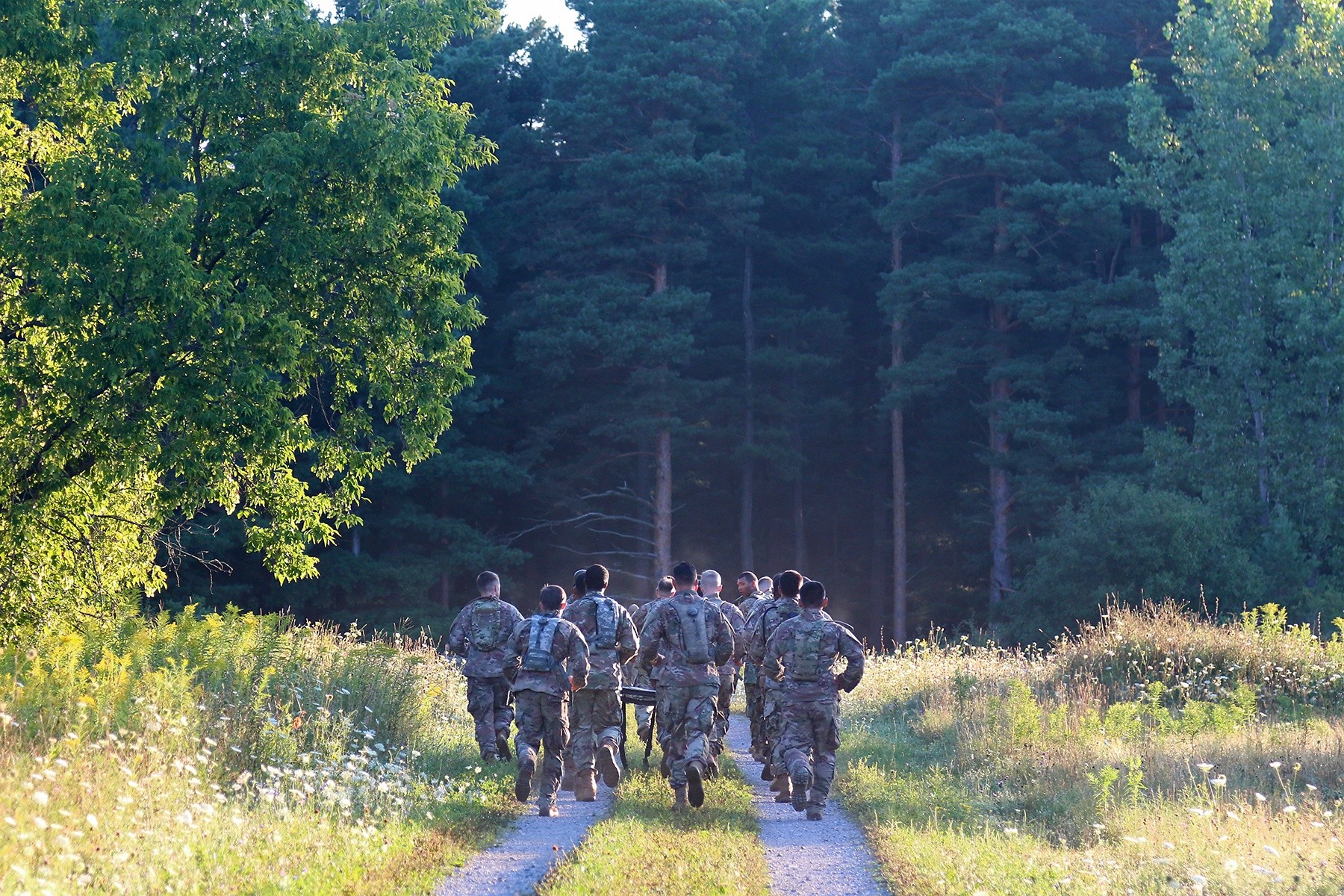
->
[522,615,560,672]
[787,618,839,682]
[471,600,501,652]
[582,595,619,649]
[663,595,717,663]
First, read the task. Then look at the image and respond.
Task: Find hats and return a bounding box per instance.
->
[574,569,585,593]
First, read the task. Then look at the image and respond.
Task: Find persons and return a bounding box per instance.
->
[561,562,864,821]
[448,571,525,764]
[501,585,591,818]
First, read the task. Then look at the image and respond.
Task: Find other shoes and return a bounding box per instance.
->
[561,773,577,792]
[485,752,498,765]
[707,754,719,778]
[749,745,767,763]
[497,732,512,762]
[639,726,650,743]
[769,778,781,792]
[762,764,775,781]
[661,740,672,779]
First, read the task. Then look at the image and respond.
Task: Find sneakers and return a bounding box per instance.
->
[775,775,792,802]
[686,761,705,808]
[673,786,686,811]
[515,759,534,802]
[599,744,620,788]
[577,769,597,801]
[807,807,823,821]
[791,767,809,812]
[539,806,559,817]
